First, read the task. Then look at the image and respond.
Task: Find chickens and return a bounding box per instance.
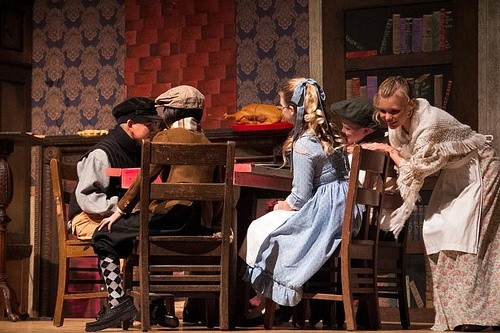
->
[223,103,287,124]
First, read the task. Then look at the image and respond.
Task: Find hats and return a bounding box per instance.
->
[330,98,375,126]
[155,85,206,109]
[112,97,163,124]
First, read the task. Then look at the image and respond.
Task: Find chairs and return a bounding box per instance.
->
[48,143,411,333]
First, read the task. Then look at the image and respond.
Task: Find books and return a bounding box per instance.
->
[347,35,365,50]
[346,76,360,99]
[367,76,377,99]
[415,73,434,106]
[360,86,367,97]
[434,74,442,109]
[442,80,453,109]
[346,51,378,58]
[380,19,392,55]
[392,9,453,54]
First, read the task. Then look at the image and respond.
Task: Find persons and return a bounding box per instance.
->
[67,96,158,240]
[359,75,500,333]
[304,97,395,324]
[239,76,362,326]
[85,85,234,332]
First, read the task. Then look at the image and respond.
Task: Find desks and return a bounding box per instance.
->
[106,165,292,332]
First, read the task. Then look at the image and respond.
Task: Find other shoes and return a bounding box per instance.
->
[85,298,138,332]
[237,307,282,327]
